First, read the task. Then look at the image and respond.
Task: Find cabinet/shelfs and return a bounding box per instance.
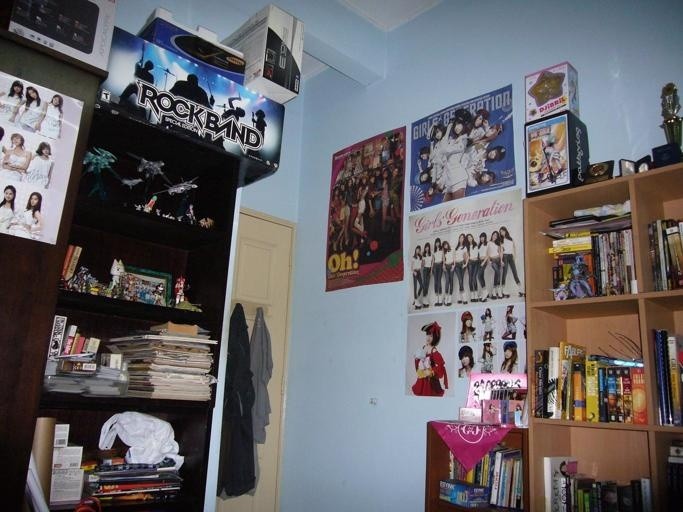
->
[0,25,244,511]
[521,163,682,511]
[425,420,529,511]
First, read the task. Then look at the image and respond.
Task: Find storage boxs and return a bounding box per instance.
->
[522,62,591,198]
[96,4,306,190]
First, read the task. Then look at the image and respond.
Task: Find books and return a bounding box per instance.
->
[647,219,682,292]
[544,456,652,512]
[43,314,219,402]
[531,341,648,425]
[61,245,82,286]
[439,442,523,509]
[81,457,183,500]
[668,440,683,464]
[653,329,683,427]
[538,200,638,299]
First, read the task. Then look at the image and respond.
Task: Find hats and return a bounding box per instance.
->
[459,346,472,360]
[462,311,472,319]
[415,109,506,205]
[503,341,517,348]
[421,322,441,334]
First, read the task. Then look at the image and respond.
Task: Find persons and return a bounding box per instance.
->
[0,80,63,240]
[458,305,518,378]
[415,109,506,209]
[412,322,448,397]
[411,226,525,310]
[328,132,402,251]
[118,61,268,157]
[473,378,523,423]
